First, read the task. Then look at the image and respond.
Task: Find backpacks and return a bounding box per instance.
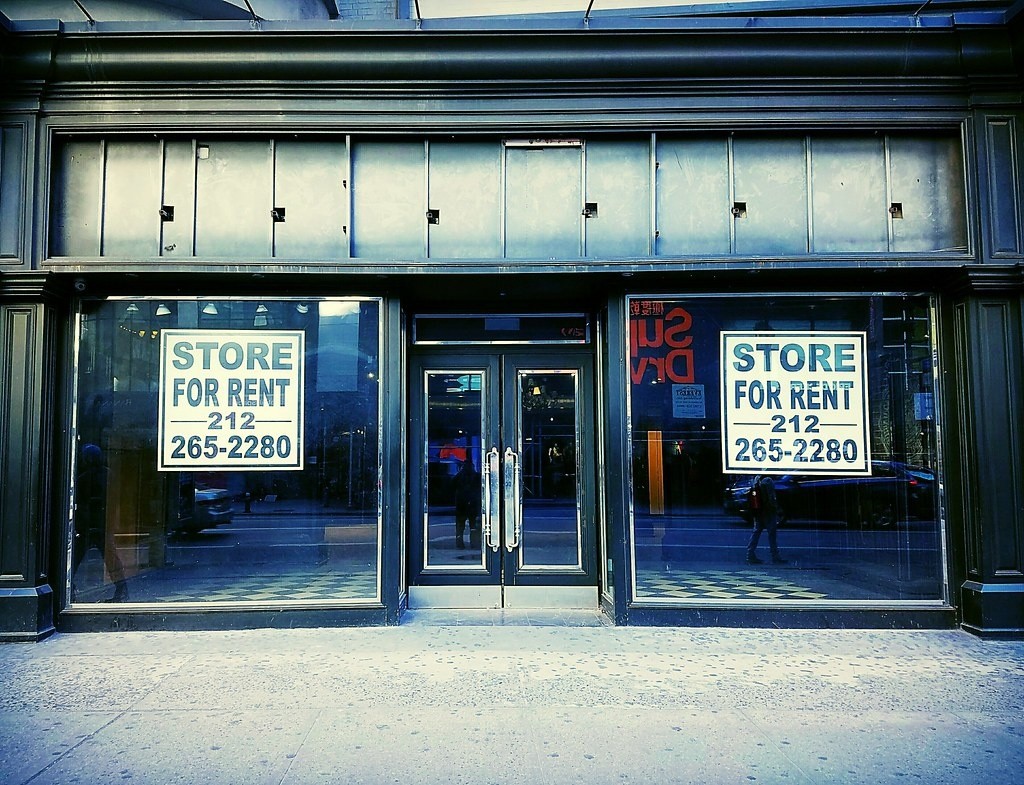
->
[747,476,768,514]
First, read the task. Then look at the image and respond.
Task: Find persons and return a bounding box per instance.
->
[71,444,127,604]
[747,478,787,566]
[452,461,481,549]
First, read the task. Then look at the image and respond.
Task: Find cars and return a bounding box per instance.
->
[163,482,234,540]
[722,461,933,532]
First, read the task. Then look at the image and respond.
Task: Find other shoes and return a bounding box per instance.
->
[773,557,789,564]
[105,580,131,603]
[746,557,762,565]
[456,541,465,549]
[470,543,482,551]
